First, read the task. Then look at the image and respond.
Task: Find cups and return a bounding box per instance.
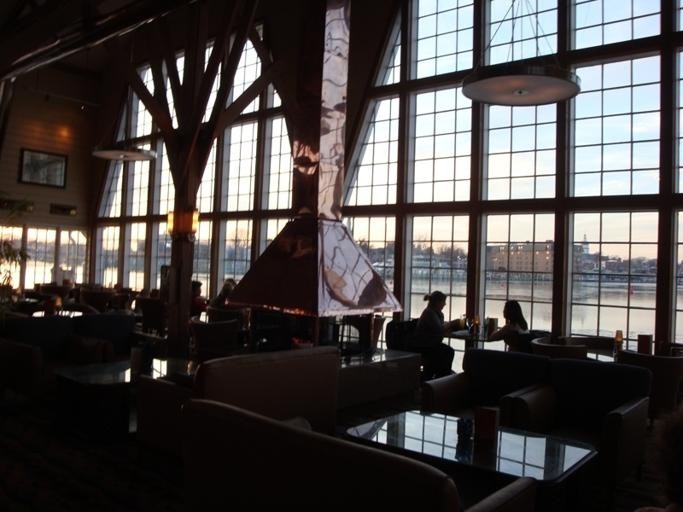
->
[474,406,497,444]
[637,333,653,355]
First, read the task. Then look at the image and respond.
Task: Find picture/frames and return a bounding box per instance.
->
[17,148,68,188]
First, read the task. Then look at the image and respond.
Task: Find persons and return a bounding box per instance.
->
[417,291,461,379]
[186,278,250,329]
[628,401,683,511]
[487,297,530,352]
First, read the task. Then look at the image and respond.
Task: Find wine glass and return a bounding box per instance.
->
[465,318,477,336]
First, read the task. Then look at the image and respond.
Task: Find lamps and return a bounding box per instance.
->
[463,0,580,107]
[92,45,157,161]
